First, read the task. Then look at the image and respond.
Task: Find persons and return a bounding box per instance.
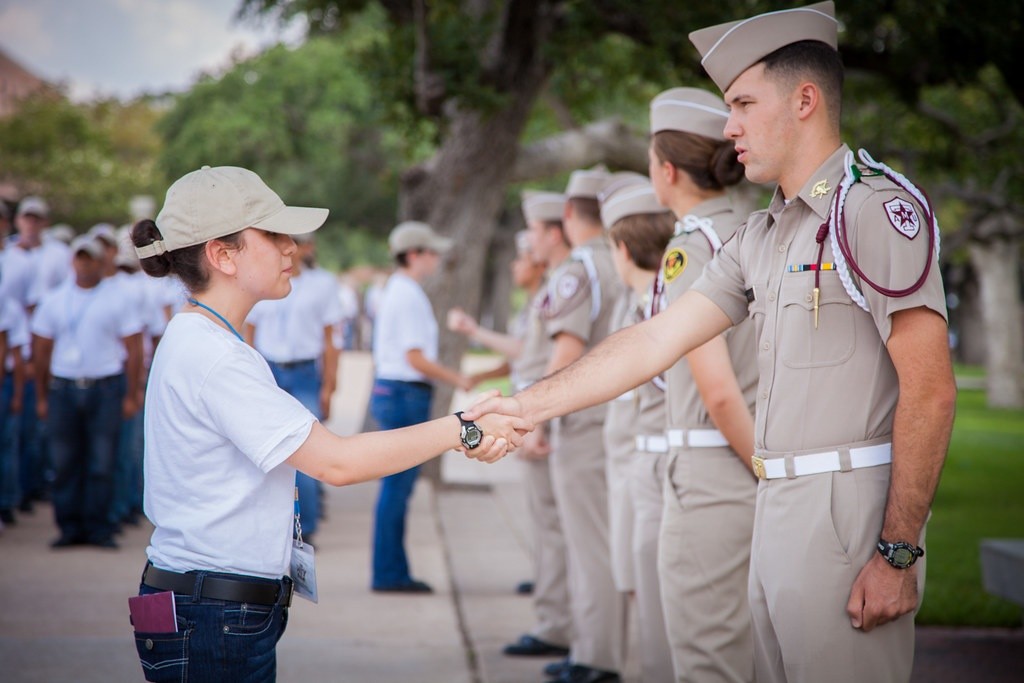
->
[445,162,676,683]
[646,87,758,683]
[128,165,536,683]
[458,0,959,683]
[0,184,385,548]
[369,218,475,594]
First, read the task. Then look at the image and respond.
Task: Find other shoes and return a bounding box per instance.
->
[545,666,619,683]
[544,661,569,676]
[503,635,569,656]
[371,581,430,593]
[517,583,531,591]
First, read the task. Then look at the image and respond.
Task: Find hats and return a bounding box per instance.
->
[649,85,734,142]
[68,234,105,261]
[87,222,116,246]
[512,229,534,250]
[131,163,330,260]
[688,0,839,94]
[387,220,454,258]
[17,195,51,220]
[112,223,144,271]
[597,169,671,229]
[520,188,566,222]
[562,163,614,202]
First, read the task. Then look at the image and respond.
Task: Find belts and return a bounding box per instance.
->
[634,434,669,452]
[751,442,893,481]
[665,427,732,447]
[142,563,294,607]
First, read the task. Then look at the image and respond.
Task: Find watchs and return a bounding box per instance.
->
[454,411,483,450]
[876,538,925,569]
